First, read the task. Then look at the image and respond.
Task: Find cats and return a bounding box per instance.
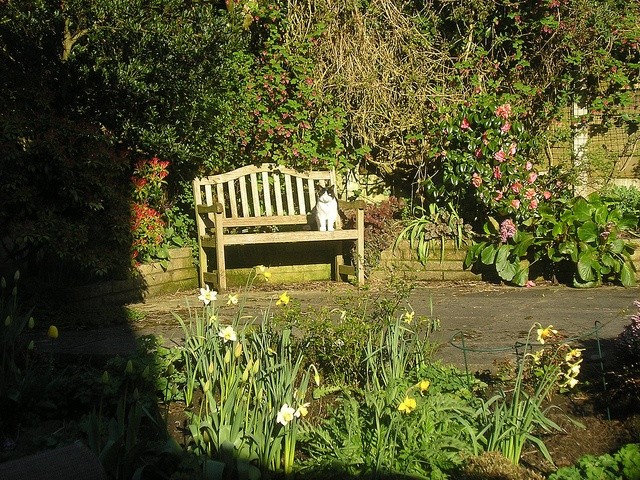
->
[315,184,339,233]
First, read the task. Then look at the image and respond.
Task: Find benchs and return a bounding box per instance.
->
[191,164,366,294]
